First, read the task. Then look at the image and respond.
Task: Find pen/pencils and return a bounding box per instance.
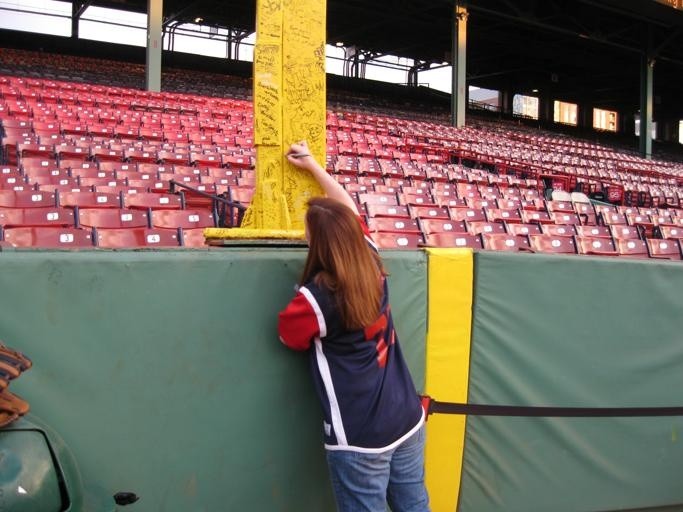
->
[285,154,311,158]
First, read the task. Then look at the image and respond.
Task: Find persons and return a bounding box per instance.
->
[277,139,432,512]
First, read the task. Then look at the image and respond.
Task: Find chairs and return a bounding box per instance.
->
[0,38,680,270]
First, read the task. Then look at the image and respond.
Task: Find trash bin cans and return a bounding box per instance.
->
[0,412,84,512]
